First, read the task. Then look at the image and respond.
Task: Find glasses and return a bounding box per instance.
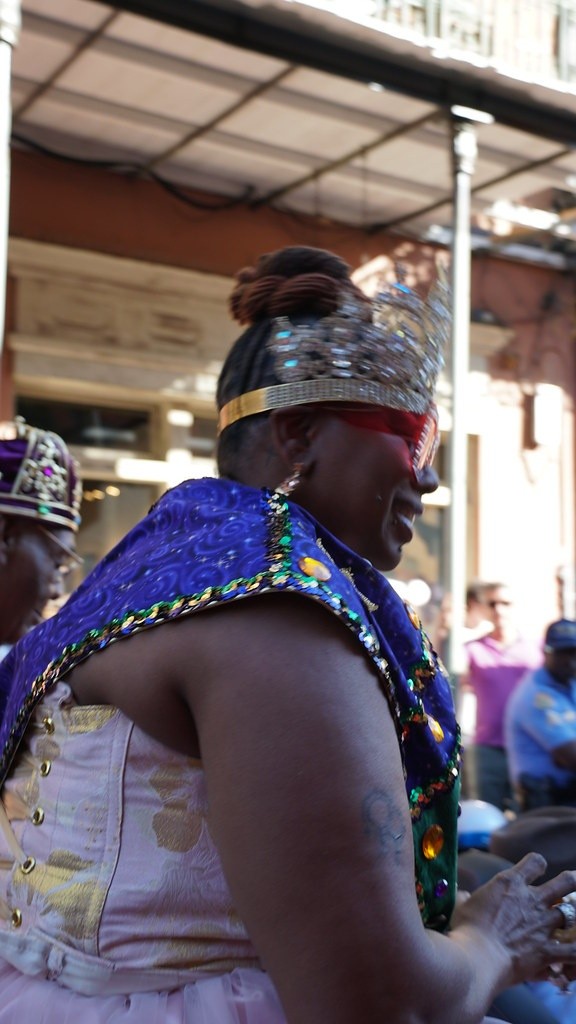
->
[487,599,511,609]
[33,522,84,582]
[305,406,441,483]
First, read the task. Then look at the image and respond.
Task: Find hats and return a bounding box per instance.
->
[546,617,576,650]
[0,415,83,533]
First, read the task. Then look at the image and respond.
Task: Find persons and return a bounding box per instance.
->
[429,579,576,817]
[0,245,576,1024]
[0,416,84,663]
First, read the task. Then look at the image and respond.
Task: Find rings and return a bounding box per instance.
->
[554,903,576,929]
